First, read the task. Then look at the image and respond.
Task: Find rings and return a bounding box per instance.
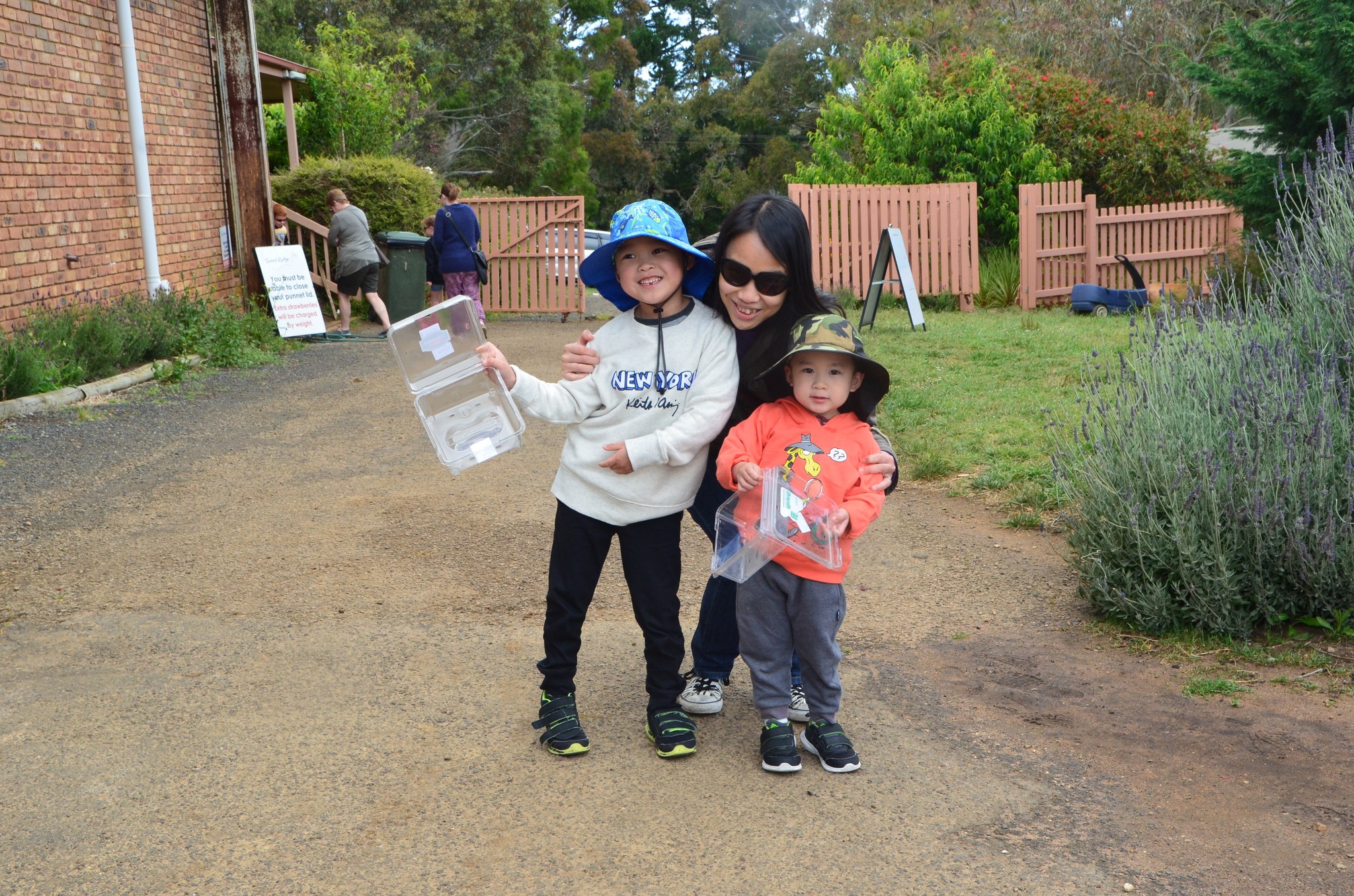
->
[884,476,892,487]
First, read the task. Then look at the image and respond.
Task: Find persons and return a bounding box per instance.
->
[716,313,885,775]
[422,214,445,308]
[272,204,288,246]
[325,188,392,338]
[559,194,900,724]
[480,199,742,760]
[433,184,488,341]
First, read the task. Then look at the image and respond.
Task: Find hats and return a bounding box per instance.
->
[579,199,717,312]
[753,314,890,421]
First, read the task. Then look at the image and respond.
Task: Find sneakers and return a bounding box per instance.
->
[678,675,723,713]
[644,709,700,757]
[785,685,811,722]
[801,720,861,773]
[377,329,389,338]
[532,688,590,755]
[759,718,803,771]
[329,330,351,335]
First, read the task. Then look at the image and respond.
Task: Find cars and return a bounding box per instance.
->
[539,225,612,284]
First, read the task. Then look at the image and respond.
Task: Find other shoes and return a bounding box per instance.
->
[480,320,487,340]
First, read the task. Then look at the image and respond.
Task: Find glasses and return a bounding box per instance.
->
[439,195,442,199]
[423,227,428,231]
[718,256,791,296]
[274,217,286,222]
[329,205,333,212]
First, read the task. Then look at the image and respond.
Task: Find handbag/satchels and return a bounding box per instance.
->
[472,250,488,285]
[375,244,390,270]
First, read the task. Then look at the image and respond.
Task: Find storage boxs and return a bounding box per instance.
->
[709,467,846,584]
[389,296,527,474]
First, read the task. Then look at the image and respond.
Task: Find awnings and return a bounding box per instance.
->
[258,48,376,105]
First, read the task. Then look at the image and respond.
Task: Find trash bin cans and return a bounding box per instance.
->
[371,231,430,326]
